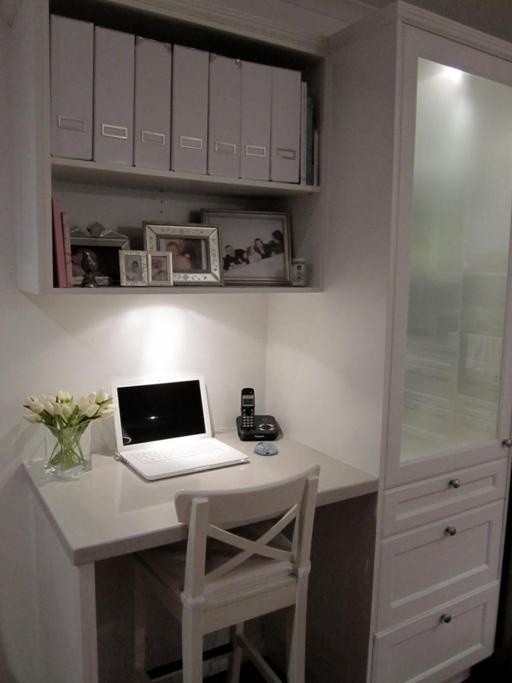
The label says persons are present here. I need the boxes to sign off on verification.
[223,230,284,270]
[168,242,191,269]
[127,260,140,279]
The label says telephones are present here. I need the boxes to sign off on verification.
[236,388,279,441]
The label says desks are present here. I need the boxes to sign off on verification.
[21,430,379,683]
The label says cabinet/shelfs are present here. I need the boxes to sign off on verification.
[17,1,331,295]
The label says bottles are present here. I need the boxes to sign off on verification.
[292,258,306,287]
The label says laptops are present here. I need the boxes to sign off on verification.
[111,375,249,482]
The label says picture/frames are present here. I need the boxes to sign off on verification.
[118,249,148,287]
[142,222,224,288]
[69,221,131,287]
[201,208,293,285]
[148,250,173,287]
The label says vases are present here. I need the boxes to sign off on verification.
[43,423,94,475]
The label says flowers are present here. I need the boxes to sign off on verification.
[25,390,116,469]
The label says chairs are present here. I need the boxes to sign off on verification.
[129,464,323,683]
